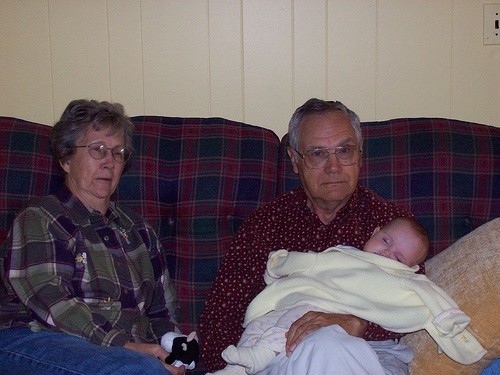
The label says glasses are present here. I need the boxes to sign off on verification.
[73,142,131,163]
[288,144,361,169]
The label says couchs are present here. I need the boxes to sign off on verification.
[0,116,500,375]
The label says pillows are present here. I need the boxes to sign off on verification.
[368,217,500,375]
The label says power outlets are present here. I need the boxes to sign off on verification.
[483,3,500,44]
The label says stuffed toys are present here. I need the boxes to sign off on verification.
[161,331,199,370]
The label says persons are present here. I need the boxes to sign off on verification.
[197,98,425,375]
[0,99,186,375]
[205,217,430,375]
